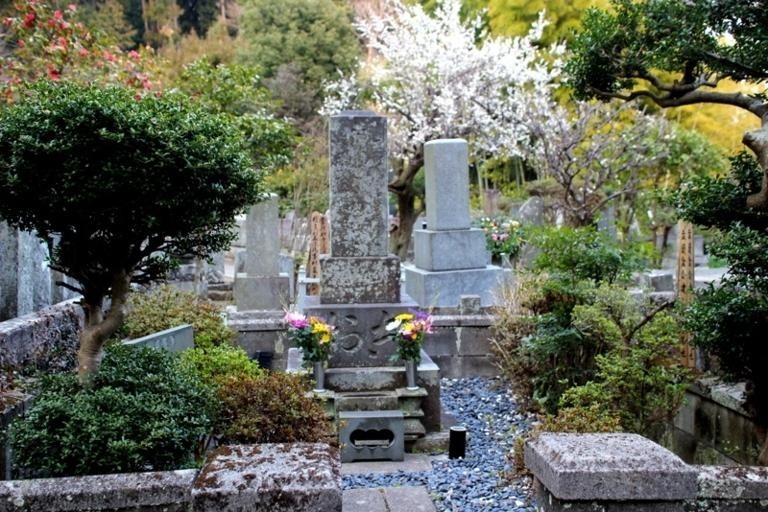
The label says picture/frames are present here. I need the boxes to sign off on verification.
[404,360,420,391]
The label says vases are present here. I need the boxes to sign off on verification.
[312,361,328,394]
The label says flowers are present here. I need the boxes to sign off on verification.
[279,306,337,370]
[382,313,434,366]
